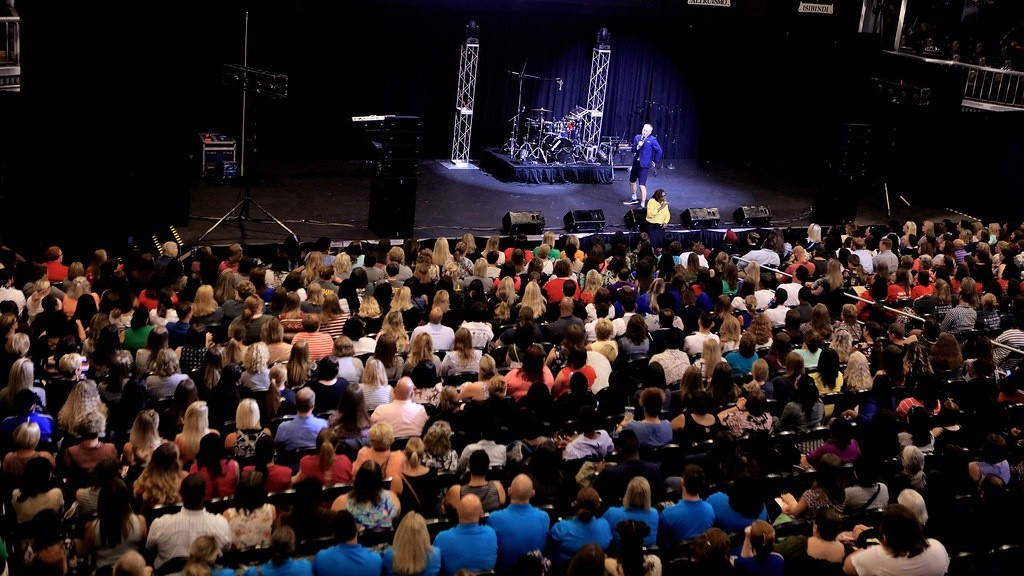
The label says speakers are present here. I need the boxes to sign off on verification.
[624,209,646,229]
[732,204,773,224]
[680,208,720,229]
[502,210,545,235]
[563,208,606,233]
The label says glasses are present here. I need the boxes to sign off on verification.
[662,193,666,198]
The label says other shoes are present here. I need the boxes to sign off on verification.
[623,197,639,205]
[637,204,645,209]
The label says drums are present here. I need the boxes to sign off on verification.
[536,121,553,133]
[540,134,574,164]
[554,121,571,133]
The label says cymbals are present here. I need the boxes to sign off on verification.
[531,108,551,112]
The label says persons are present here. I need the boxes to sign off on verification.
[623,124,662,209]
[0,218,1024,575]
[902,18,1024,105]
[645,189,670,251]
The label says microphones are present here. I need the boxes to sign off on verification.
[559,80,562,90]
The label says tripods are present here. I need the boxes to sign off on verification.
[515,106,549,166]
[196,12,301,242]
